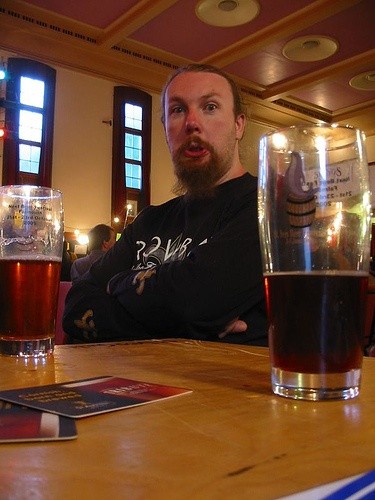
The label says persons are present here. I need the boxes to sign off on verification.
[60,64,337,347]
[71,224,115,280]
[43,238,77,282]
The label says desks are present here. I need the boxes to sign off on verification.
[0,338,375,500]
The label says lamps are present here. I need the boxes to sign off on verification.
[349,71,375,91]
[282,35,340,63]
[195,0,261,28]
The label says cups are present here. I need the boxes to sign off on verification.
[0,185,64,357]
[256,124,370,402]
